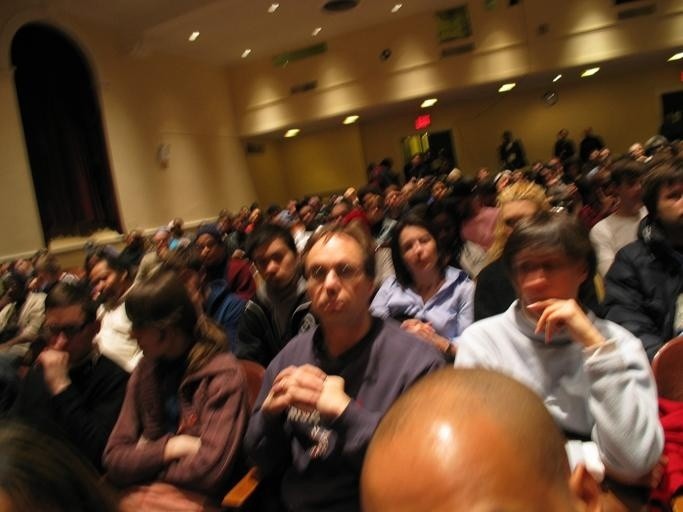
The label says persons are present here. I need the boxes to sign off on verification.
[1,124,681,511]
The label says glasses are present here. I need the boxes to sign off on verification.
[44,319,87,336]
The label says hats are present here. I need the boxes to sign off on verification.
[194,224,221,244]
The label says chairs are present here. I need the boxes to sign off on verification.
[222,361,267,507]
[649,337,683,398]
